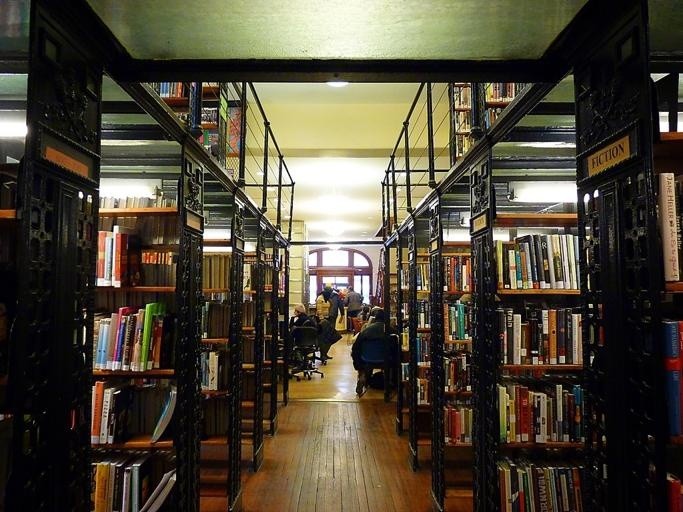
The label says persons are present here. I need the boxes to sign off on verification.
[288,280,398,375]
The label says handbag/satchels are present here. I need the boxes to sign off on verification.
[337,316,346,330]
[369,369,384,390]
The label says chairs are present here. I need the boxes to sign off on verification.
[355,329,400,401]
[290,326,325,381]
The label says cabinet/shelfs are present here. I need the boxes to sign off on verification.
[203,180,243,512]
[152,81,201,140]
[92,140,202,511]
[572,0,682,510]
[407,222,428,476]
[393,236,407,437]
[201,81,227,180]
[428,191,469,512]
[469,152,582,512]
[264,234,278,434]
[472,82,529,135]
[278,243,290,406]
[244,217,264,473]
[446,83,471,165]
[0,0,104,512]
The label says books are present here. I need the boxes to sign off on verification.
[388,79,683,512]
[0,80,286,512]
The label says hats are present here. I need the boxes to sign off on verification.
[324,287,333,293]
[295,303,306,312]
